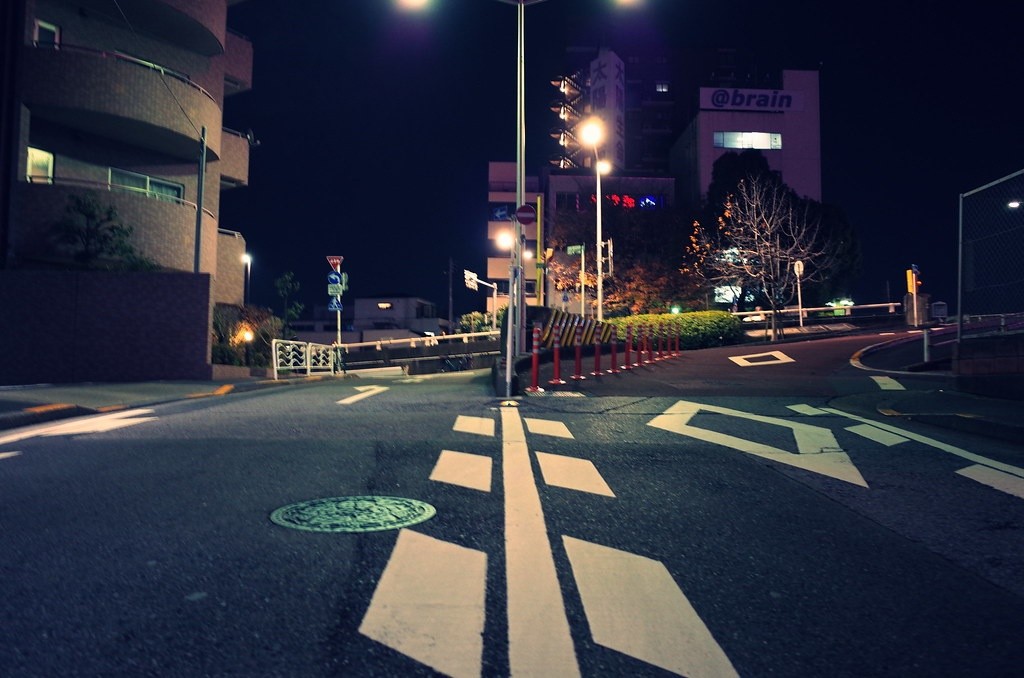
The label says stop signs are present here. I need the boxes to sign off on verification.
[515,204,536,230]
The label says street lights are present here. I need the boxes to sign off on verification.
[582,121,605,323]
[241,253,251,307]
[400,0,642,352]
